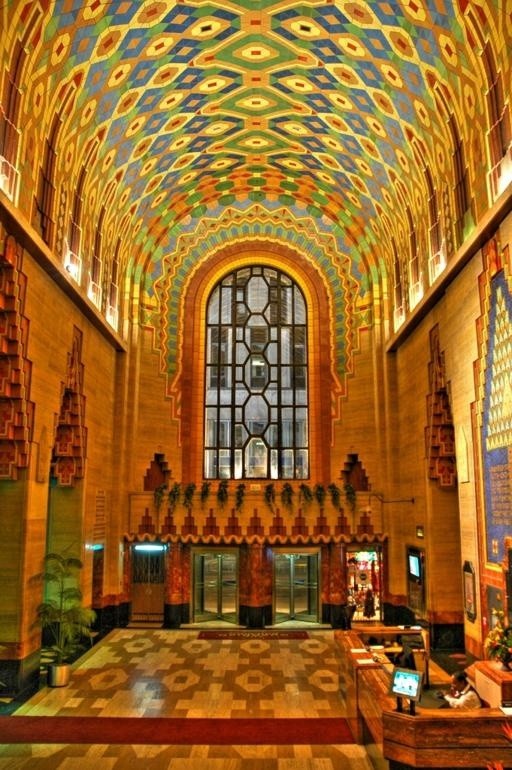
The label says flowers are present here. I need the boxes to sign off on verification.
[483,607,512,663]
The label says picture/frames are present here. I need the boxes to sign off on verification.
[463,560,477,623]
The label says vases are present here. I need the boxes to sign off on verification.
[496,653,512,671]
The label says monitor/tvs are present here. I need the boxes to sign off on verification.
[408,548,423,584]
[387,668,424,701]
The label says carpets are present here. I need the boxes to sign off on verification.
[197,630,310,640]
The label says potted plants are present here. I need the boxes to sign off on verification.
[26,539,98,688]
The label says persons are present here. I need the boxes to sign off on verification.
[442,672,483,709]
